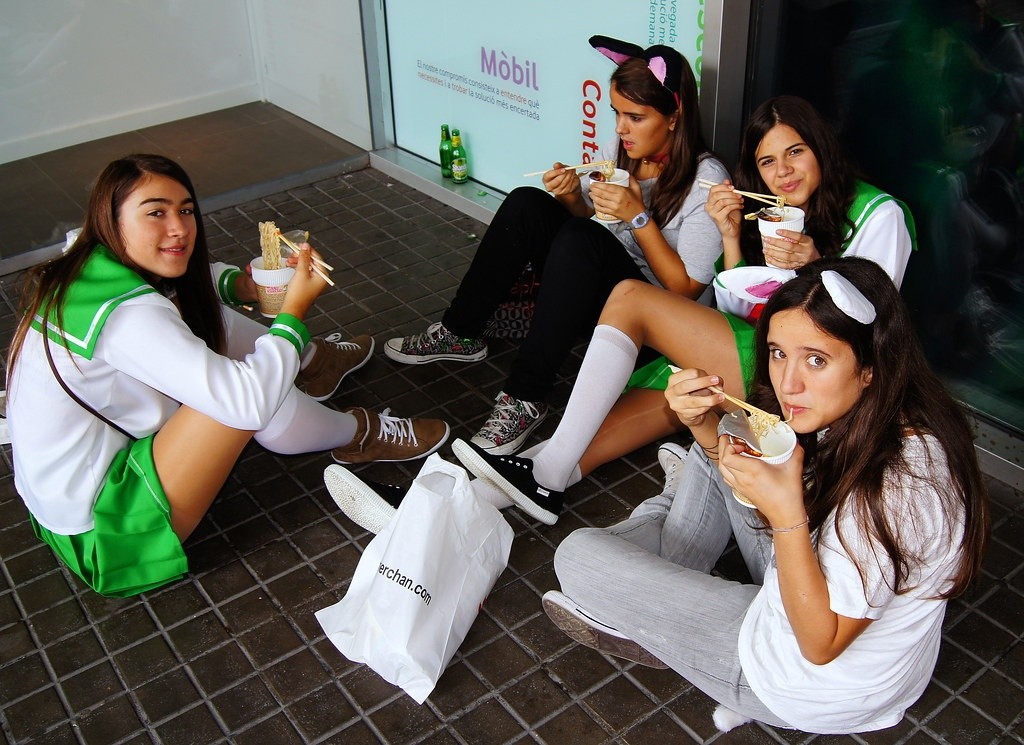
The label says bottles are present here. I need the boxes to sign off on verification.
[439,124,453,178]
[449,129,468,184]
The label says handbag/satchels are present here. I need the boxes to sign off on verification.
[482,263,540,339]
[316,453,516,705]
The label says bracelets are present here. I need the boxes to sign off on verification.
[773,516,809,533]
[697,442,719,460]
[629,210,650,230]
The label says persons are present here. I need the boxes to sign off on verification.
[4,153,450,599]
[875,0,1024,346]
[541,257,985,735]
[384,35,730,454]
[325,97,917,536]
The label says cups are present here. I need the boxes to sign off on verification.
[588,169,631,224]
[759,206,805,266]
[731,416,797,508]
[250,257,295,315]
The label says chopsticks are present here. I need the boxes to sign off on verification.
[668,365,780,426]
[698,178,782,207]
[277,234,336,288]
[524,160,610,178]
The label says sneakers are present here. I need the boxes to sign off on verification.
[470,391,549,455]
[657,443,689,502]
[383,322,488,364]
[324,464,408,535]
[541,590,670,669]
[451,437,566,525]
[331,407,450,464]
[300,333,375,400]
